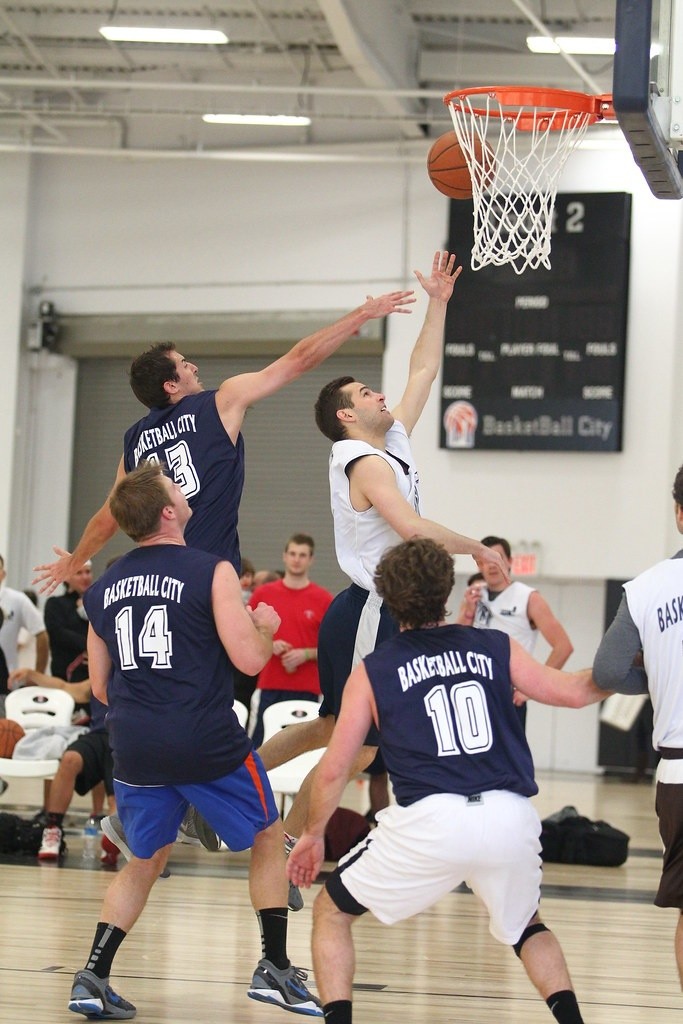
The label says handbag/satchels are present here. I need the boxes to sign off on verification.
[540,803,631,867]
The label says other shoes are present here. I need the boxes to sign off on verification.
[82,822,98,837]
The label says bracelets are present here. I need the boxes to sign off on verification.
[465,610,474,619]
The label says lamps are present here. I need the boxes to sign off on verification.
[93,12,228,46]
[201,99,315,127]
[523,15,663,59]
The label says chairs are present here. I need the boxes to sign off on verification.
[260,698,332,821]
[229,698,250,733]
[0,687,76,780]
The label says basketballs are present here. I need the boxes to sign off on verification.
[427,129,499,201]
[0,718,25,759]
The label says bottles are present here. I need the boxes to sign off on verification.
[82,813,99,864]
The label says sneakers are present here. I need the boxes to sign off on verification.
[99,834,120,867]
[285,840,304,911]
[174,817,198,842]
[39,826,61,859]
[66,968,137,1019]
[247,959,322,1015]
[99,813,170,879]
[193,805,222,851]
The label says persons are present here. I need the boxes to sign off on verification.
[592,465,683,1000]
[68,467,325,1021]
[457,536,574,738]
[0,556,126,865]
[192,250,512,911]
[285,538,644,1024]
[467,572,487,587]
[239,534,389,822]
[32,291,416,879]
